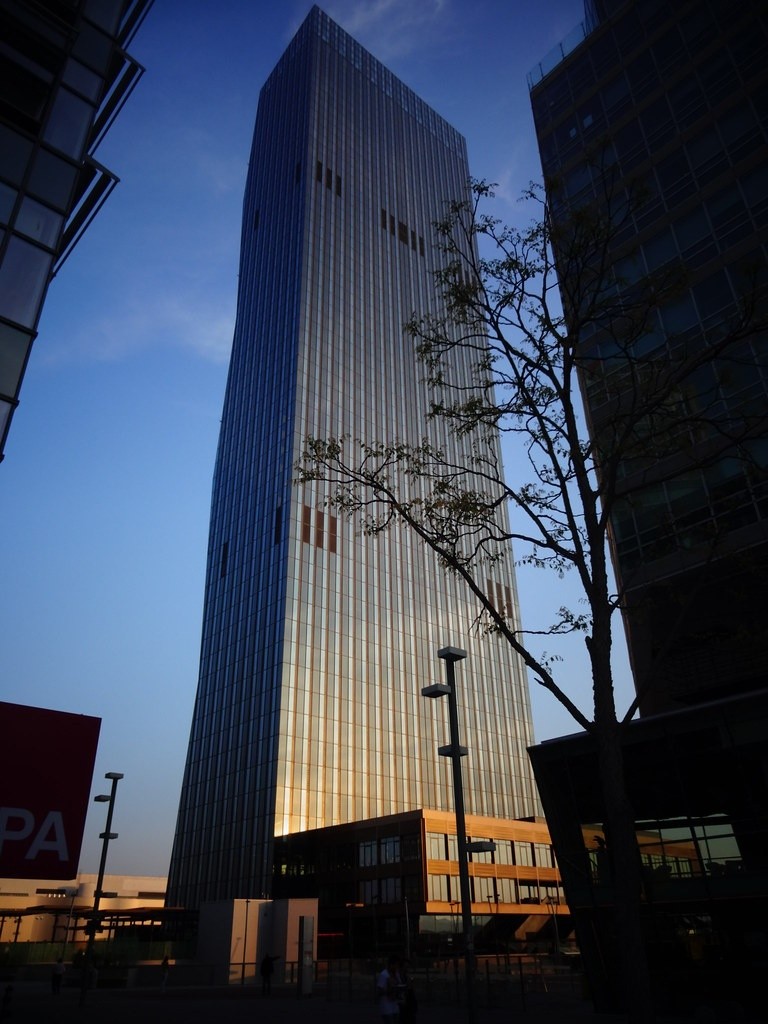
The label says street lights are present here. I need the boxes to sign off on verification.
[59,888,80,968]
[371,894,383,973]
[240,898,250,984]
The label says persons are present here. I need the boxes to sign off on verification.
[51,958,66,995]
[260,953,281,997]
[159,955,169,990]
[377,955,418,1024]
[302,951,313,1000]
